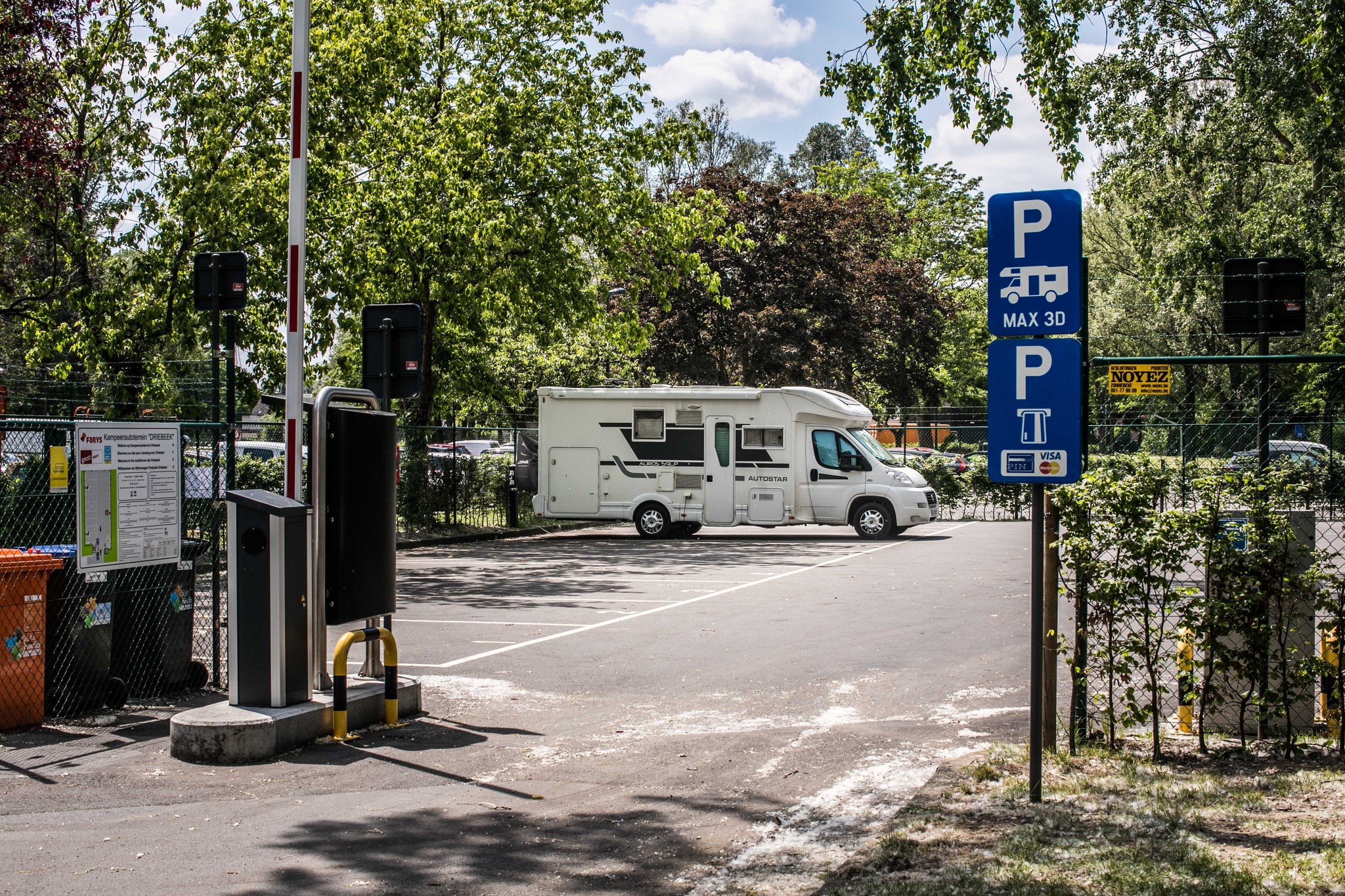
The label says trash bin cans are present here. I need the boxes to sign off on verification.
[1203,510,1315,735]
[111,537,209,696]
[225,489,312,707]
[15,545,128,718]
[0,547,64,733]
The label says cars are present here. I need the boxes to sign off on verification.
[1215,438,1345,505]
[884,446,988,483]
[399,439,515,489]
[184,439,309,469]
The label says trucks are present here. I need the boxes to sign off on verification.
[511,383,939,541]
